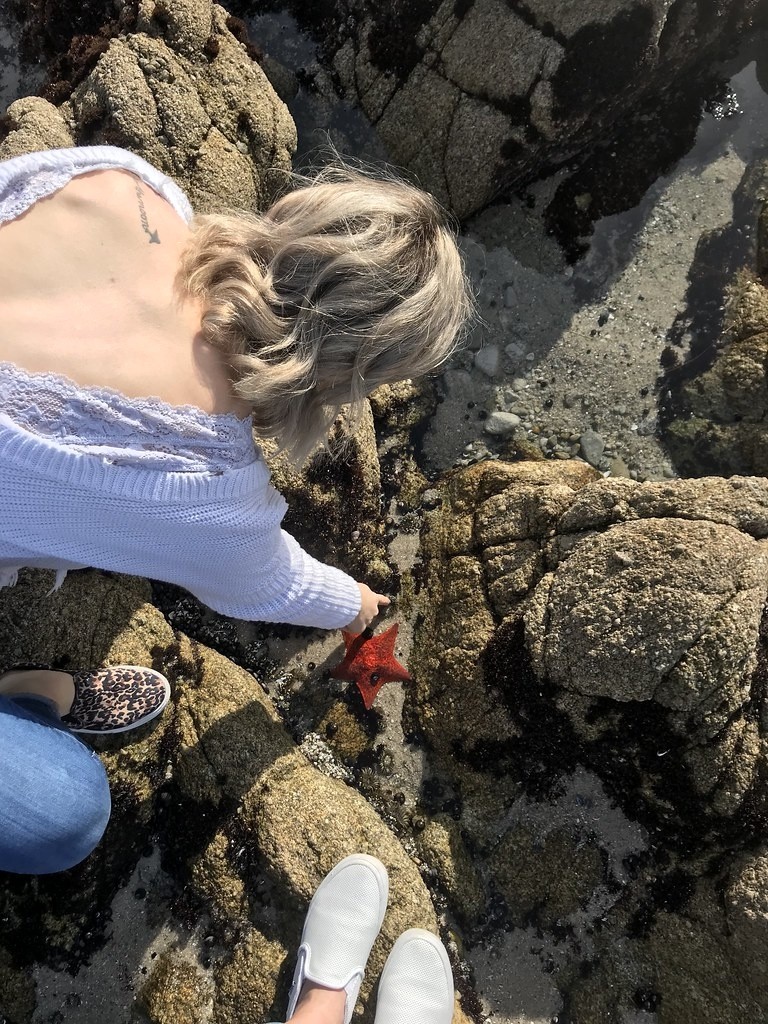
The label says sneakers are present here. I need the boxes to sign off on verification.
[286,853,389,1024]
[0,665,171,733]
[373,928,455,1024]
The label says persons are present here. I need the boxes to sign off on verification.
[284,852,453,1024]
[0,146,489,872]
